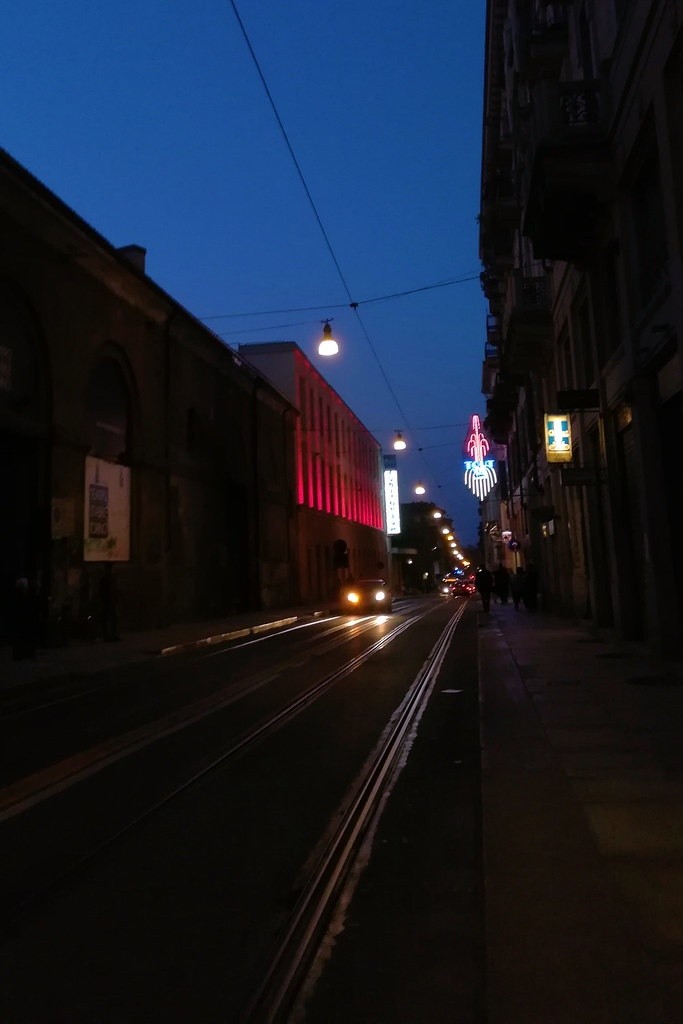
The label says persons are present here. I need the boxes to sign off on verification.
[13,577,38,660]
[475,563,493,611]
[512,564,540,613]
[494,561,510,604]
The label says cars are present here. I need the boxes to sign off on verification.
[347,579,392,615]
[441,577,477,598]
